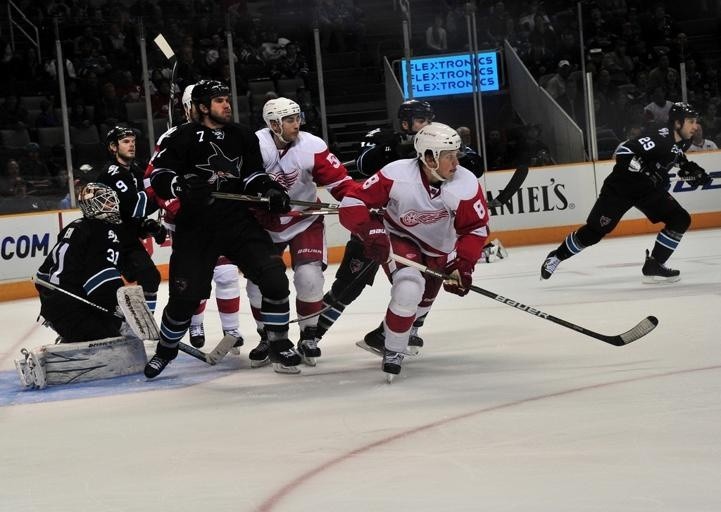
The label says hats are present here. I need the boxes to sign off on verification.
[558,60,570,68]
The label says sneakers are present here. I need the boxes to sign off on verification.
[223,329,243,348]
[480,248,489,262]
[382,348,405,374]
[20,348,47,389]
[409,325,423,346]
[483,242,502,258]
[188,323,204,348]
[13,358,34,390]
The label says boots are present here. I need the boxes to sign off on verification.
[142,355,168,379]
[541,250,563,280]
[642,249,680,276]
[364,321,385,353]
[247,324,322,375]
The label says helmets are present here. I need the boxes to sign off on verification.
[262,97,305,126]
[413,121,462,154]
[397,100,436,120]
[78,183,122,224]
[181,85,195,111]
[181,85,194,106]
[668,103,702,122]
[191,79,231,107]
[105,126,136,147]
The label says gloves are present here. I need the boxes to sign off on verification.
[359,221,391,264]
[173,173,211,204]
[443,256,476,297]
[139,217,166,245]
[644,167,670,189]
[264,188,290,215]
[678,162,712,189]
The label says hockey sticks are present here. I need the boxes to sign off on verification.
[213,165,529,216]
[391,254,659,345]
[31,275,236,366]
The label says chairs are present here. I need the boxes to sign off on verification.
[1,0,721,159]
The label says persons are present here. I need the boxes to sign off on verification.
[541,103,712,285]
[316,100,490,380]
[93,126,166,313]
[1,0,720,216]
[142,80,243,355]
[249,96,353,369]
[144,81,302,379]
[34,183,161,344]
